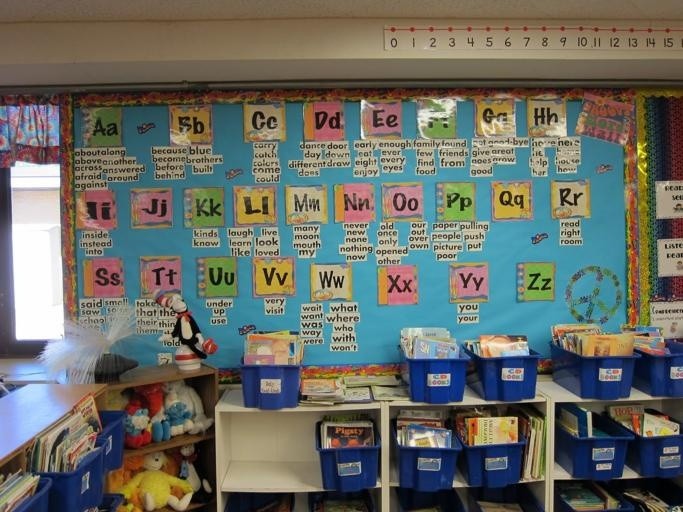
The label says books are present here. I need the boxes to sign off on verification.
[299,374,412,407]
[244,330,304,365]
[0,394,103,512]
[397,403,547,481]
[465,335,530,358]
[320,413,375,449]
[551,324,672,358]
[400,328,460,359]
[479,501,523,512]
[559,403,683,512]
[313,491,368,512]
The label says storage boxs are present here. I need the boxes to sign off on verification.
[4,407,128,512]
[212,335,682,511]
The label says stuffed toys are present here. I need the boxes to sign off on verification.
[154,288,217,371]
[108,379,213,512]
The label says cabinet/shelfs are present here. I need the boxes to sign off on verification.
[0,381,114,511]
[107,357,220,511]
